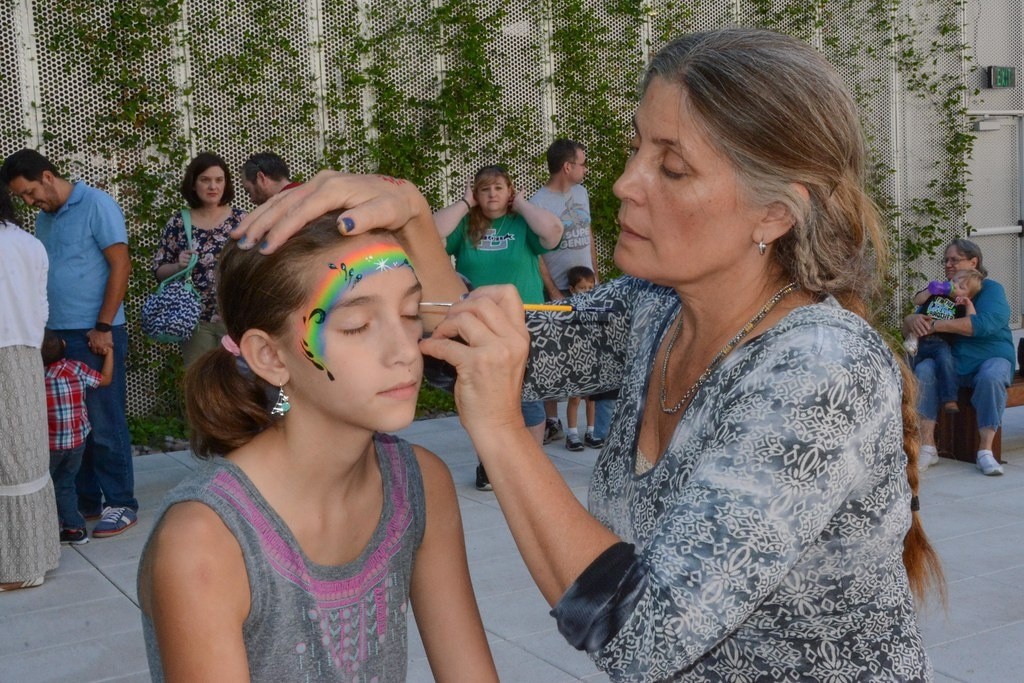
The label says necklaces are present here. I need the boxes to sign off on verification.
[489,216,507,241]
[659,283,802,414]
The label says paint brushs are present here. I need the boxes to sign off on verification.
[419,302,572,311]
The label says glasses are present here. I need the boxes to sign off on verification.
[566,159,587,169]
[941,255,972,267]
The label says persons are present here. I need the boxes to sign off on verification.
[240,151,305,206]
[0,178,61,591]
[152,151,248,368]
[230,28,935,683]
[433,140,617,490]
[3,149,139,544]
[136,208,501,683]
[901,239,1024,475]
[41,336,113,543]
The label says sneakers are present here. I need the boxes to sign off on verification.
[917,444,939,472]
[566,432,584,451]
[543,417,564,444]
[60,526,89,545]
[944,400,959,414]
[81,504,103,521]
[93,505,138,537]
[476,461,494,491]
[584,434,604,449]
[977,454,1004,476]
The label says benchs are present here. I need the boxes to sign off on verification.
[934,374,1024,465]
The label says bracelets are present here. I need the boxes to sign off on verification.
[462,197,471,212]
[95,321,113,332]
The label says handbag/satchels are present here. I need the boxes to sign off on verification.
[139,208,203,343]
